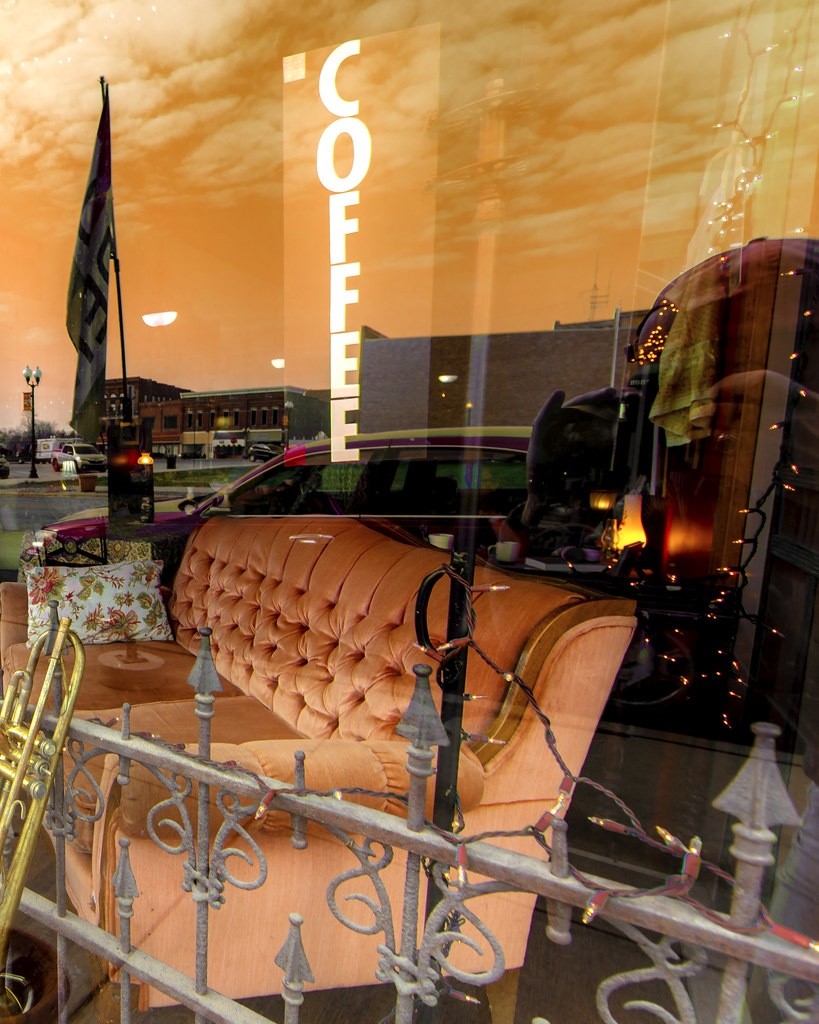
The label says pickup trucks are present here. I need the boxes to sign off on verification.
[51,444,107,473]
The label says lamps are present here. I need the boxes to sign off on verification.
[138,453,155,465]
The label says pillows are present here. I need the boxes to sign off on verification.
[23,559,174,650]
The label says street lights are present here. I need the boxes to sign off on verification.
[23,365,42,478]
[467,400,472,431]
[285,401,293,451]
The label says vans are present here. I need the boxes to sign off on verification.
[36,438,85,464]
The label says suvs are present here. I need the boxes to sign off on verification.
[248,444,284,463]
[20,427,535,565]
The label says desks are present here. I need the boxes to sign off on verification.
[17,516,205,582]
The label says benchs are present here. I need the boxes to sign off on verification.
[0,515,639,1024]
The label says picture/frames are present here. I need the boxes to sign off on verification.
[119,415,141,448]
